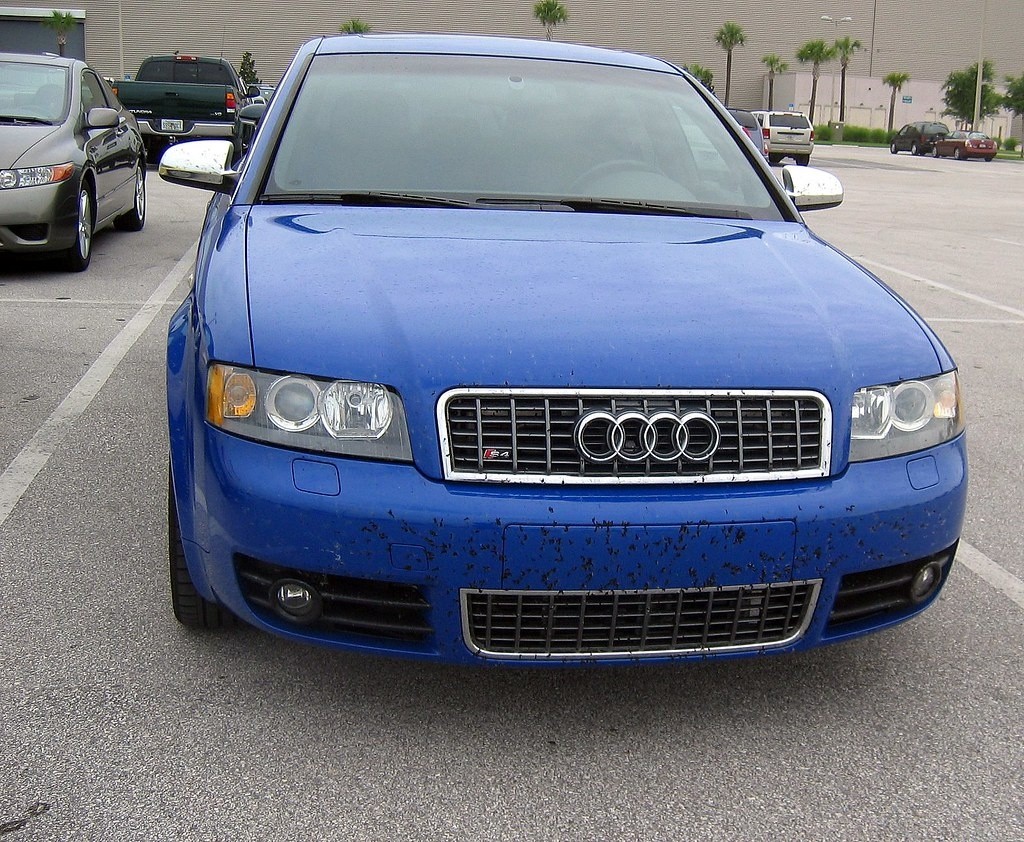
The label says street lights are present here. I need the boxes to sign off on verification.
[820,16,852,121]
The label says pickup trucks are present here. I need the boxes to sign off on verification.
[103,55,261,165]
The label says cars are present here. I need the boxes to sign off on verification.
[930,130,997,162]
[247,84,276,105]
[890,121,949,156]
[726,108,769,164]
[0,52,148,273]
[751,110,814,167]
[158,32,969,667]
